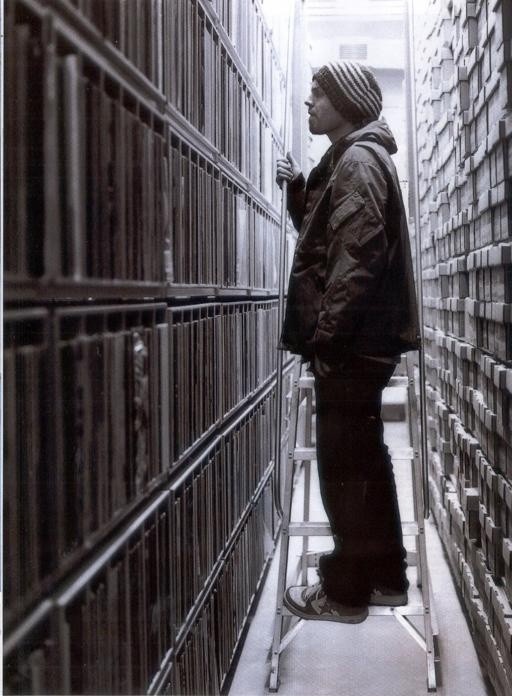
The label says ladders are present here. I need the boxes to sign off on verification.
[268,352,438,694]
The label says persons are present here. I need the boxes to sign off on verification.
[276,58,423,625]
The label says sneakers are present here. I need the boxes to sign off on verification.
[282,583,368,624]
[371,585,410,608]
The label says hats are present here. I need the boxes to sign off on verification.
[312,61,383,122]
